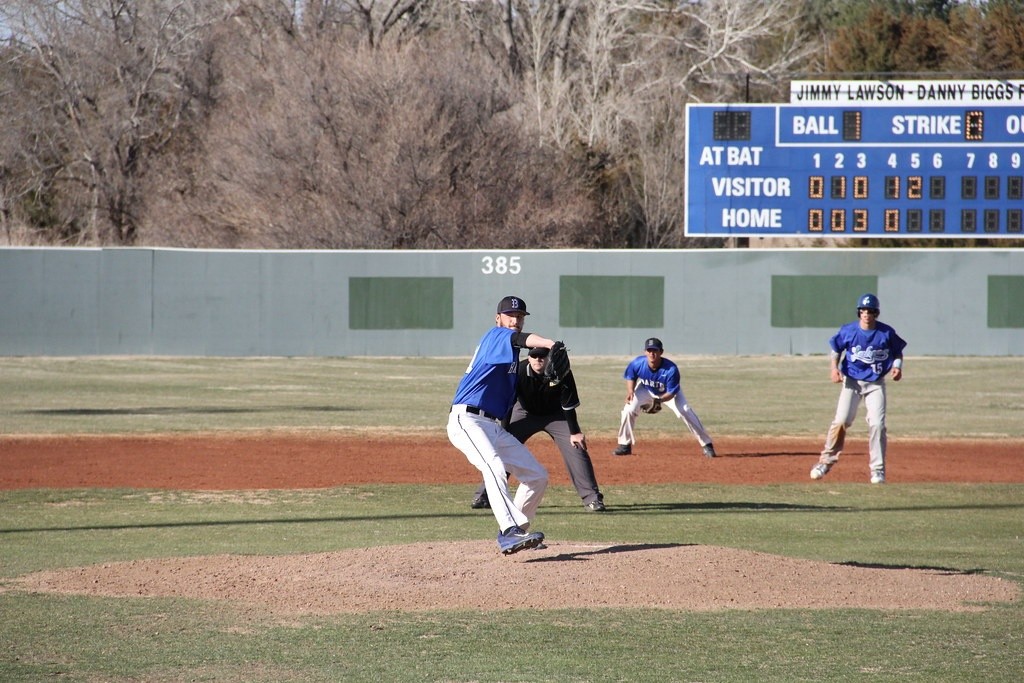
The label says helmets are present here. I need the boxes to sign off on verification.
[857,293,879,310]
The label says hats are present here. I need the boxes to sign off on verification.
[528,348,549,357]
[644,338,662,351]
[497,296,531,316]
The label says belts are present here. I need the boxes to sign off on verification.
[449,404,505,428]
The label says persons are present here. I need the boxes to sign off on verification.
[612,338,717,457]
[446,294,570,554]
[809,294,908,483]
[470,347,605,511]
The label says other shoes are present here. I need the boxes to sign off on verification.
[703,447,715,457]
[613,446,631,455]
[809,461,833,480]
[870,472,885,484]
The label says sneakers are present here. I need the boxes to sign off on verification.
[497,530,546,549]
[497,527,544,555]
[588,501,605,511]
[470,499,490,509]
